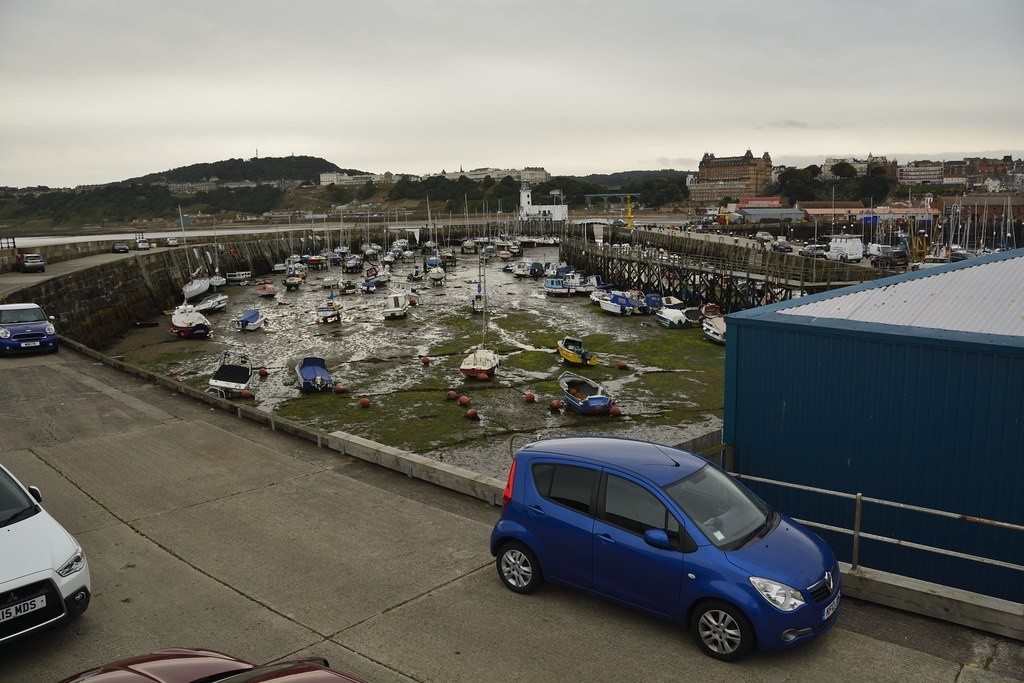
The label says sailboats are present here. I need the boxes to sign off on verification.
[317,237,344,317]
[460,255,500,377]
[178,194,559,315]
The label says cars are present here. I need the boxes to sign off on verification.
[134,239,149,250]
[59,648,367,682]
[756,231,976,271]
[613,218,641,227]
[0,303,58,356]
[15,254,45,273]
[0,463,90,646]
[489,436,841,662]
[163,238,179,247]
[111,242,129,253]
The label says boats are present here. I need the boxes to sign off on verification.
[557,336,597,365]
[171,299,212,339]
[209,350,253,396]
[501,260,727,344]
[231,308,267,331]
[558,370,614,415]
[295,357,334,393]
